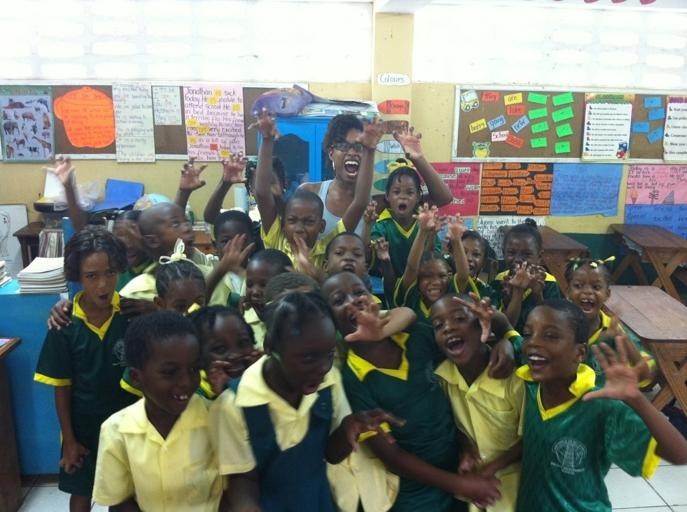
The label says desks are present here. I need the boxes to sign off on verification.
[602,285,686,423]
[182,221,211,254]
[494,224,589,300]
[2,264,106,479]
[14,222,88,267]
[0,334,22,506]
[609,221,687,304]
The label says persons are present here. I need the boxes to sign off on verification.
[30,107,685,511]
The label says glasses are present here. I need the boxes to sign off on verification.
[330,142,363,152]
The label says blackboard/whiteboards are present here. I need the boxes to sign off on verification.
[1,205,31,277]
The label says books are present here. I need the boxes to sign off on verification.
[0,258,13,290]
[17,257,67,294]
